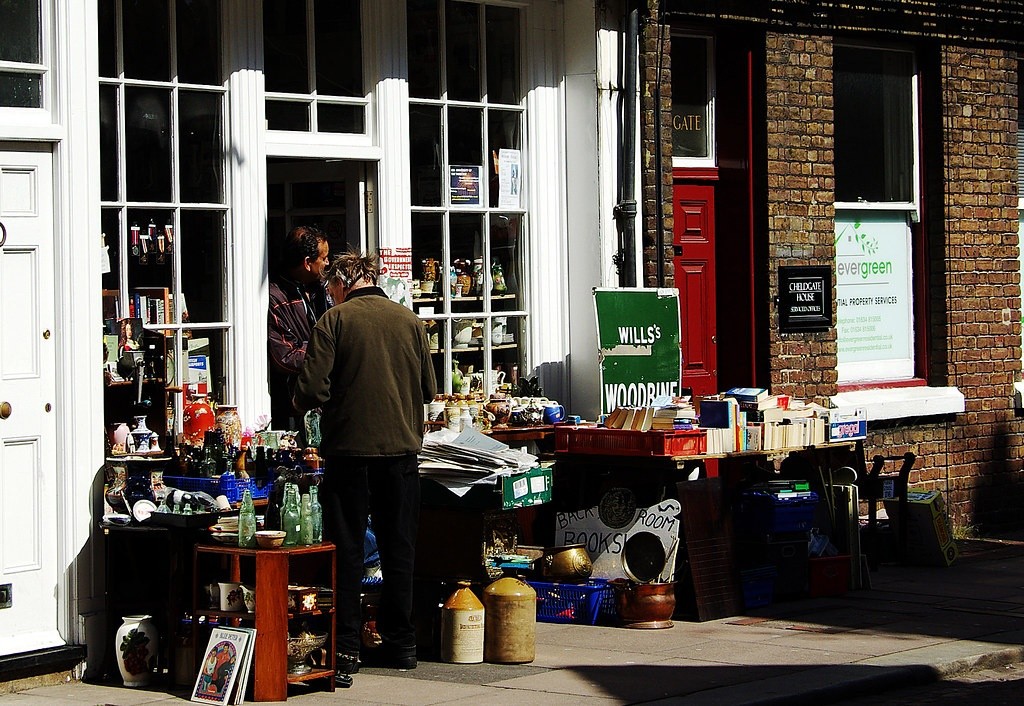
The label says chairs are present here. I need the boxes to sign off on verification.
[854,452,917,572]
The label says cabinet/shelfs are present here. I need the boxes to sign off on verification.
[195,541,337,702]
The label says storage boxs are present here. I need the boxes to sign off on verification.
[883,488,959,568]
[556,426,708,455]
[819,407,867,443]
[528,580,621,626]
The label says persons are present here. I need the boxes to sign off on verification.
[119,319,140,351]
[268,223,437,669]
[118,318,145,352]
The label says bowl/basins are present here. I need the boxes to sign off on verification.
[239,585,256,613]
[608,578,676,629]
[548,542,593,582]
[254,531,286,548]
[621,530,666,582]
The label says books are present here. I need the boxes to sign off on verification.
[604,388,826,456]
[129,286,174,325]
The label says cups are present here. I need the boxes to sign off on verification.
[510,397,565,428]
[218,582,243,612]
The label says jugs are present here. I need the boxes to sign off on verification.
[479,370,505,394]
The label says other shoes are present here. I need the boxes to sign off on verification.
[308,638,362,673]
[364,645,418,668]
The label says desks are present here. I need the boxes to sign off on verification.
[99,475,521,685]
[538,440,860,624]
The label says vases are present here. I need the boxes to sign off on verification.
[548,543,593,585]
[606,580,678,629]
[116,615,159,687]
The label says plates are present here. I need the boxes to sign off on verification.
[211,533,239,545]
[133,500,157,521]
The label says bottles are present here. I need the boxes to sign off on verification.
[165,432,318,486]
[441,580,484,663]
[183,504,192,515]
[281,483,322,546]
[158,500,167,513]
[484,567,536,664]
[173,505,180,514]
[433,393,484,433]
[238,488,257,547]
